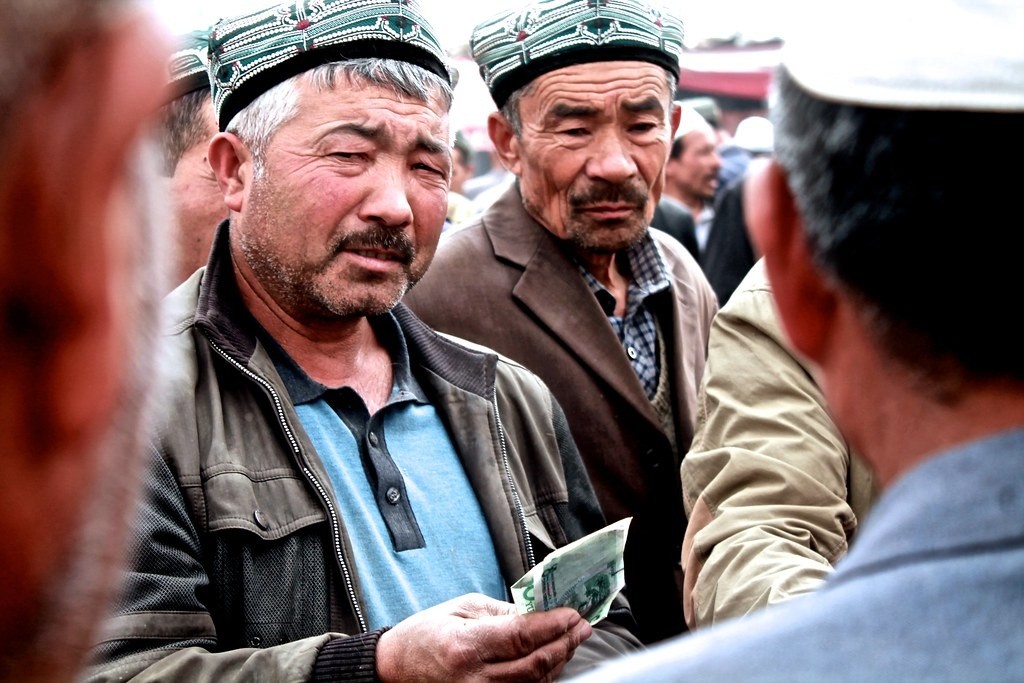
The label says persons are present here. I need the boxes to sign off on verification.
[651,97,772,308]
[398,0,722,649]
[566,15,1024,683]
[679,252,881,634]
[119,18,229,304]
[440,127,516,233]
[63,0,644,683]
[0,1,178,682]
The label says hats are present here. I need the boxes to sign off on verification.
[733,116,776,151]
[469,0,684,108]
[667,99,712,141]
[768,0,1024,117]
[150,28,213,112]
[207,0,450,131]
